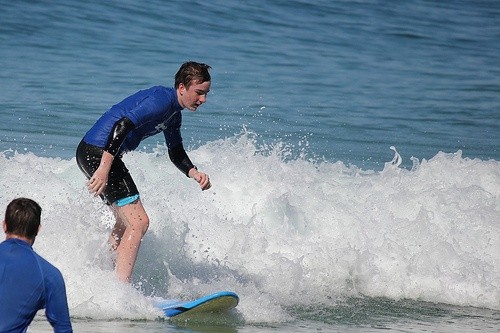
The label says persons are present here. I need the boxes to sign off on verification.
[76,61,213,284]
[0,197,73,333]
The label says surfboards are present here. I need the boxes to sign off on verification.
[149,292,240,319]
